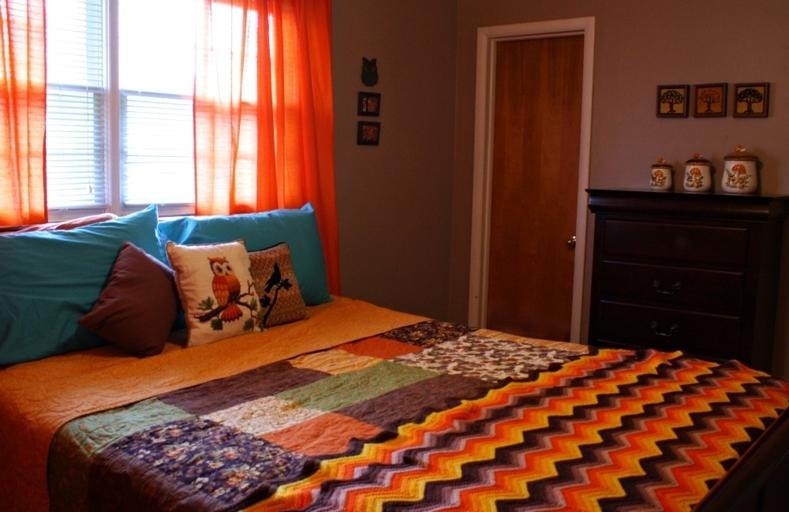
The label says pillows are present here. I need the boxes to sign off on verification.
[165,239,268,348]
[0,202,169,365]
[74,240,183,358]
[15,212,119,233]
[158,201,335,305]
[247,241,311,328]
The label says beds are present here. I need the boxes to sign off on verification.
[0,294,789,512]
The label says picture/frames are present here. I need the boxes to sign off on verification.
[357,91,381,116]
[656,84,690,119]
[733,82,770,118]
[357,120,381,145]
[692,82,728,118]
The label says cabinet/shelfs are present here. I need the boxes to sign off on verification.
[584,187,789,373]
